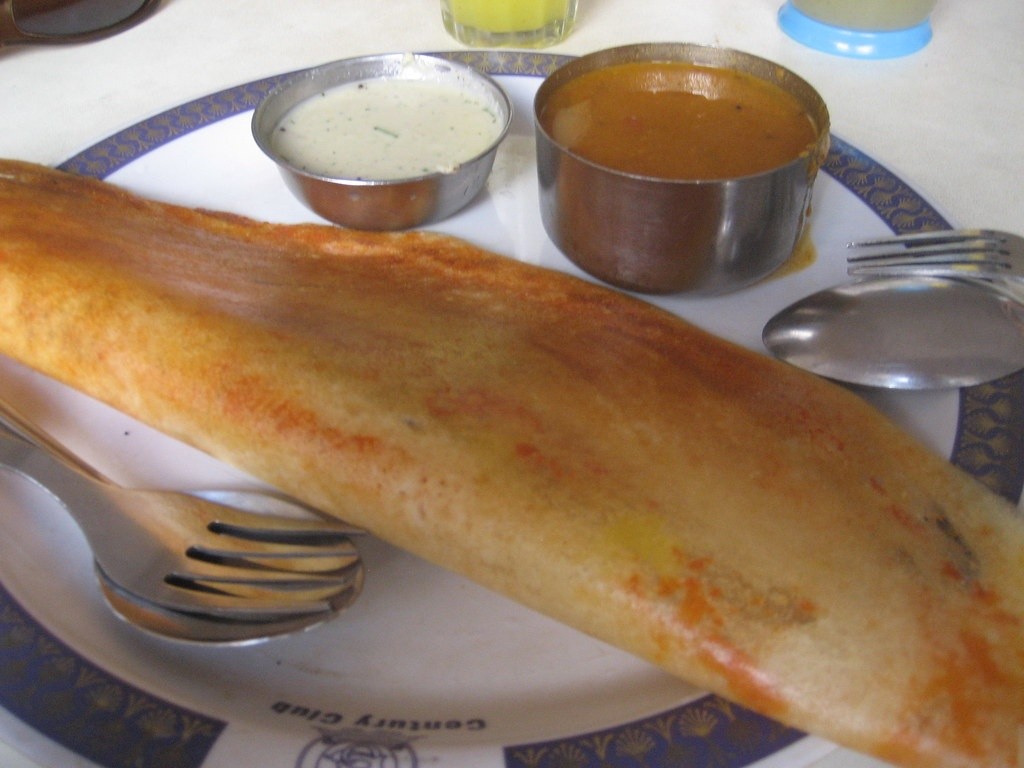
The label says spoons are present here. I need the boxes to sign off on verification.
[762,275,1024,390]
[0,400,366,646]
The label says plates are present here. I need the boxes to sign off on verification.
[0,52,1024,768]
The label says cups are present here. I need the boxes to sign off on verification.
[777,0,939,58]
[440,0,577,50]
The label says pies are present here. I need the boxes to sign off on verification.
[1,156,1024,768]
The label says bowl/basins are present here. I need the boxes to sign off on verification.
[532,43,830,297]
[252,53,513,231]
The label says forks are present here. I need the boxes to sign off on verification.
[843,225,1024,280]
[0,427,366,617]
